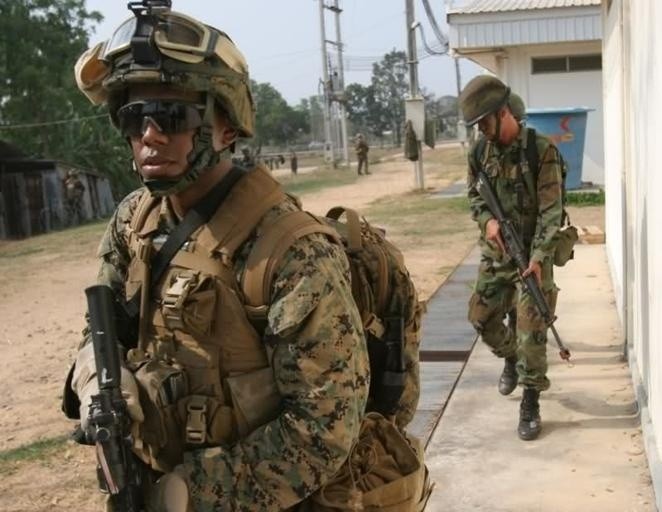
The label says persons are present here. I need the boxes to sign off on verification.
[64,168,89,225]
[355,132,372,176]
[461,72,564,442]
[240,145,255,166]
[289,147,298,175]
[61,0,436,511]
[505,91,569,334]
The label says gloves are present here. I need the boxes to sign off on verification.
[149,473,192,511]
[79,366,145,440]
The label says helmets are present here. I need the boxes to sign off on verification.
[101,14,253,139]
[457,76,511,127]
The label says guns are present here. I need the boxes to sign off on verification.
[85,284,142,512]
[472,171,571,364]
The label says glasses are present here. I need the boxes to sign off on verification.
[102,12,210,65]
[115,98,207,139]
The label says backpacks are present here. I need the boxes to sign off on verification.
[239,206,421,429]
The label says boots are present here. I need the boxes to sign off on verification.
[498,355,518,395]
[519,390,540,440]
